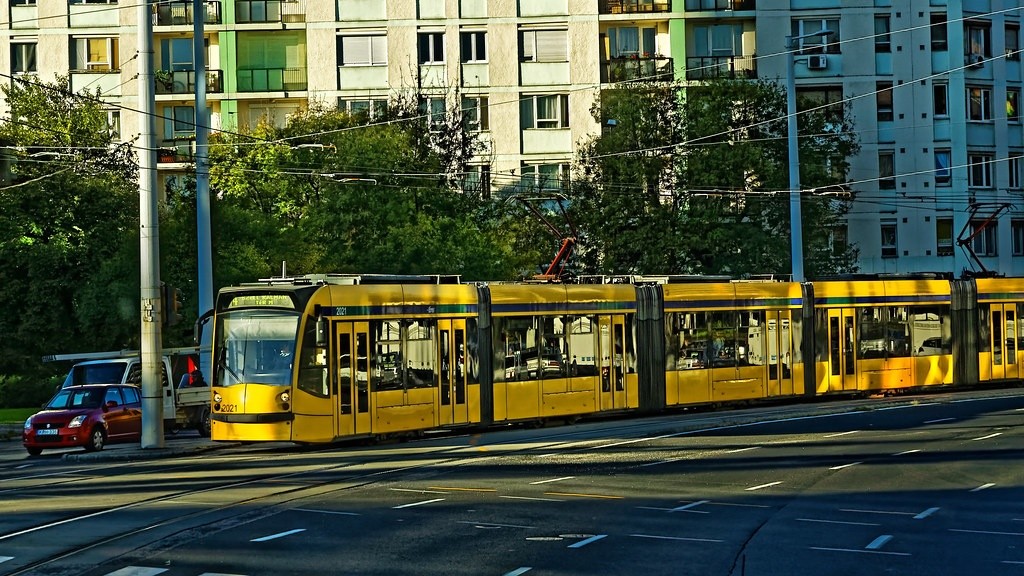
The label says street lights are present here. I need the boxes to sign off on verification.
[785,30,837,284]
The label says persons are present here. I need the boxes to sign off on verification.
[190,368,208,388]
[272,341,295,371]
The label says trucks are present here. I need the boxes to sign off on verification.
[58,354,212,439]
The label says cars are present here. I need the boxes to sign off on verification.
[21,383,142,456]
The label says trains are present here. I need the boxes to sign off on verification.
[191,255,1024,443]
